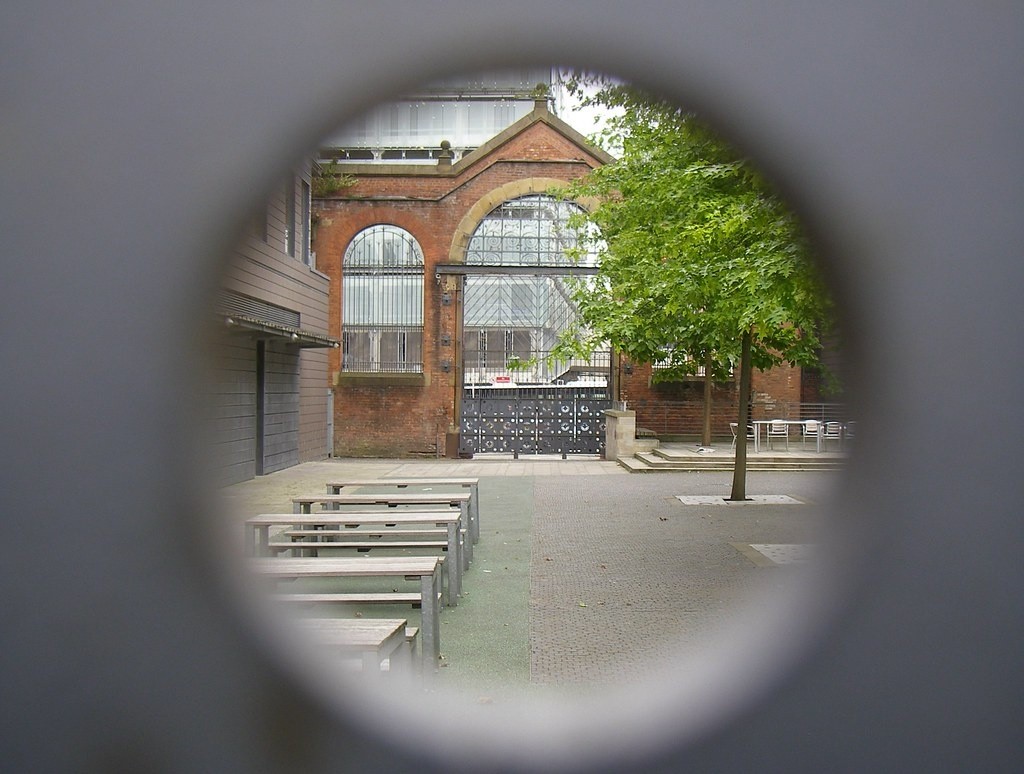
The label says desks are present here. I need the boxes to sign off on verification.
[282,618,409,702]
[752,421,822,453]
[325,478,480,546]
[292,493,474,569]
[240,554,441,680]
[246,511,462,606]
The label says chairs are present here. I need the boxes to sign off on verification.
[767,420,789,451]
[824,422,843,452]
[844,421,856,452]
[730,423,754,453]
[803,420,824,451]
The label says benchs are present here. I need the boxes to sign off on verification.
[257,502,479,670]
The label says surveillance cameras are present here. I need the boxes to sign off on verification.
[435,274,442,279]
